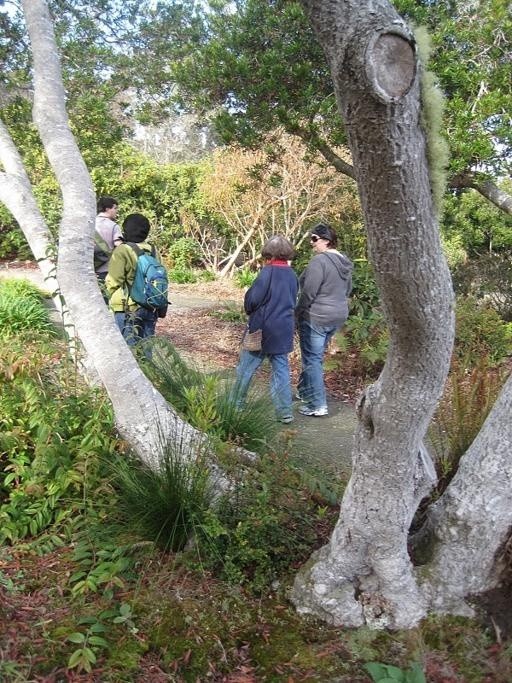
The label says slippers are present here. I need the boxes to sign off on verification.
[119,242,168,311]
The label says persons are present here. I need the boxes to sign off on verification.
[104,214,165,368]
[94,198,125,284]
[295,221,358,419]
[228,230,299,423]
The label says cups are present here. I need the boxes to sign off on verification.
[310,235,323,242]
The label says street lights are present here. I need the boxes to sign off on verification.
[242,328,262,351]
[94,230,112,268]
[158,305,167,318]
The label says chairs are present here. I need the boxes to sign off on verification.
[295,391,328,416]
[273,414,294,423]
